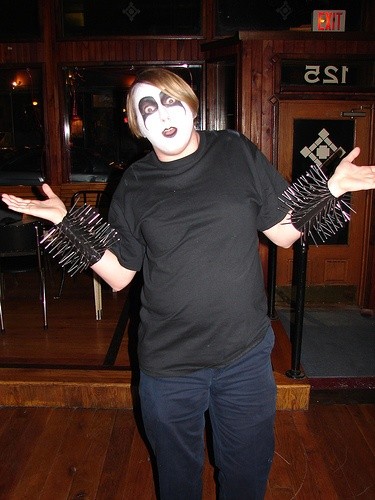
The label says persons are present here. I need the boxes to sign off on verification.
[1,68,375,500]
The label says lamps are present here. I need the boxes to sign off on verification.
[71,114,82,137]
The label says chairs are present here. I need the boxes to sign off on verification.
[0,190,108,335]
